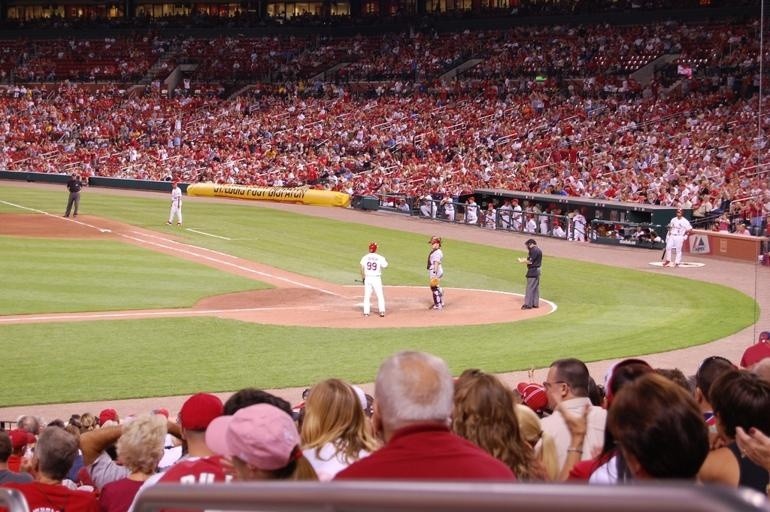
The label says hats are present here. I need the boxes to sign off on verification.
[526,239,535,244]
[100,409,116,426]
[7,430,27,448]
[205,403,301,471]
[429,236,440,244]
[181,394,224,429]
[517,383,548,410]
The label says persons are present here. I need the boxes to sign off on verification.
[517,239,542,309]
[1,331,770,511]
[1,0,769,265]
[425,235,445,309]
[360,243,388,318]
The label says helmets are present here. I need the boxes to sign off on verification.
[369,244,376,253]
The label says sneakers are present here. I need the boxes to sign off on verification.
[433,304,441,309]
[521,305,530,309]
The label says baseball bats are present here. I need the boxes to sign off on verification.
[661,249,665,260]
[354,279,363,283]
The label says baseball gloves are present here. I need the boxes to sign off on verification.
[430,277,440,288]
